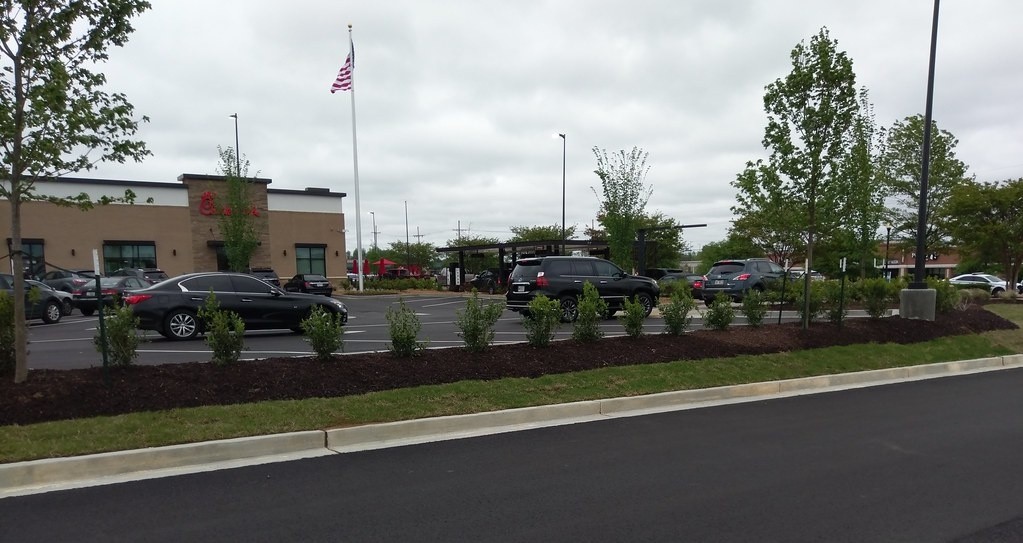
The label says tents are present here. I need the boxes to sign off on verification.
[373,258,396,272]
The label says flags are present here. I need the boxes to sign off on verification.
[330,40,355,93]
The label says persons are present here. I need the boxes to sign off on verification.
[399,265,421,275]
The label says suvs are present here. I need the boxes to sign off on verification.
[41,270,104,317]
[244,267,281,290]
[114,267,170,286]
[704,258,798,309]
[506,256,661,323]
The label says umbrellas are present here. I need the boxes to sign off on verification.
[353,258,357,274]
[363,259,369,274]
[379,257,386,278]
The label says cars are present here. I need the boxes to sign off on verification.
[121,271,347,340]
[938,273,1023,298]
[283,274,332,296]
[0,273,73,323]
[470,268,510,295]
[74,274,154,316]
[655,273,703,300]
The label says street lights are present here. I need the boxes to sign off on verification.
[230,112,240,176]
[884,222,892,281]
[559,133,566,255]
